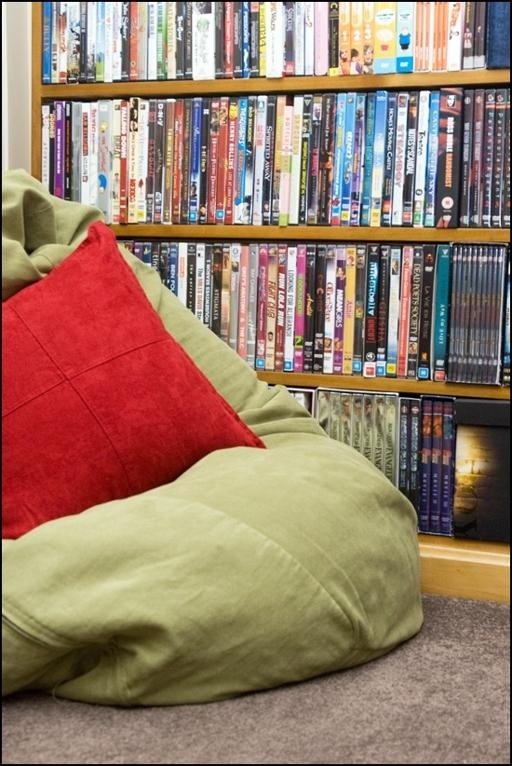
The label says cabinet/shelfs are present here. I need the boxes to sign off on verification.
[30,0,509,603]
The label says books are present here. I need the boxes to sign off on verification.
[122,242,512,377]
[287,390,512,544]
[38,87,509,229]
[43,1,511,83]
[113,238,511,389]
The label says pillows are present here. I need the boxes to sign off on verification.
[0,216,266,543]
